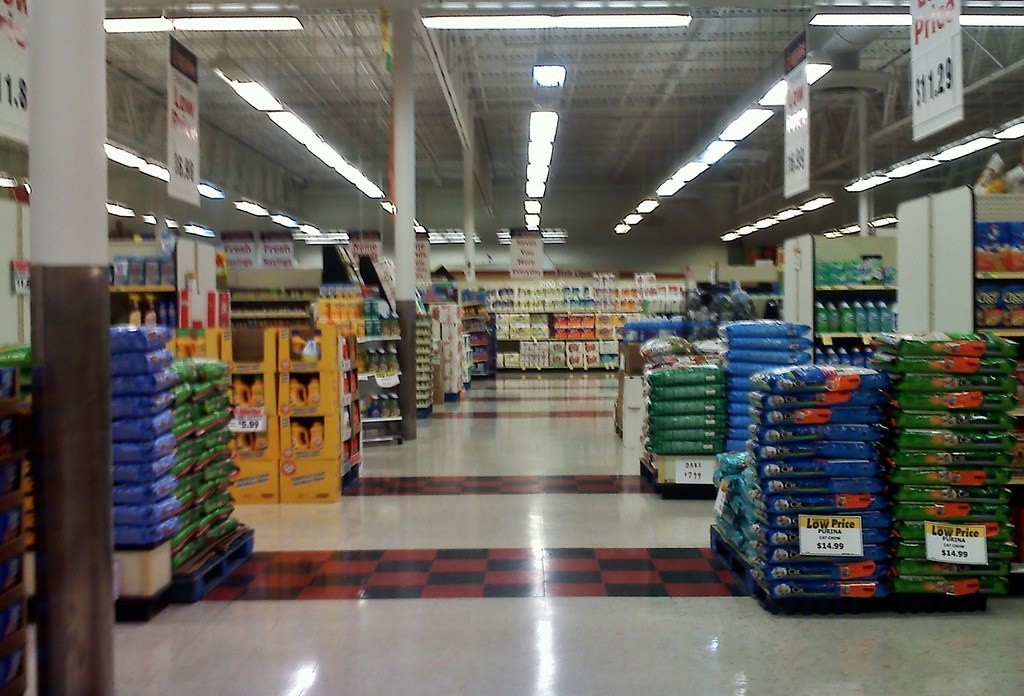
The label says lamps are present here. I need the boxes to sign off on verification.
[104,15,304,32]
[524,112,559,231]
[421,15,692,28]
[808,12,1024,28]
[614,0,832,235]
[532,51,566,90]
[844,91,1024,191]
[211,54,385,199]
[720,194,835,241]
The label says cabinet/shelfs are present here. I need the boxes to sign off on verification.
[974,270,1024,576]
[416,313,434,420]
[228,286,403,443]
[465,315,498,381]
[814,284,898,338]
[488,312,683,370]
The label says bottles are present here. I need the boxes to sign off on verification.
[307,378,321,408]
[313,330,321,348]
[287,374,307,408]
[317,282,401,418]
[126,292,176,326]
[251,376,264,407]
[302,340,319,362]
[816,347,876,367]
[815,300,896,333]
[290,329,306,363]
[290,421,309,452]
[309,421,325,453]
[231,375,251,408]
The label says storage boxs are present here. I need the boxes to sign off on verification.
[167,324,364,503]
[428,305,466,393]
[611,340,642,433]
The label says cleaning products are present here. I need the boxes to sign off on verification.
[290,421,325,450]
[815,346,875,369]
[289,376,320,407]
[234,429,269,452]
[232,374,265,408]
[814,299,898,334]
